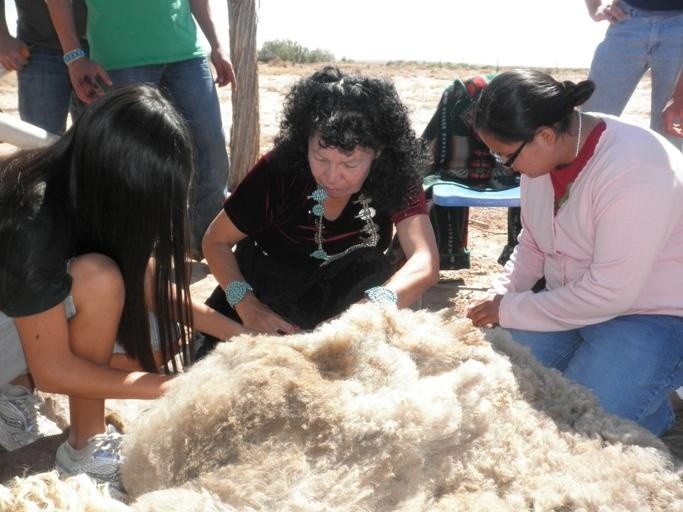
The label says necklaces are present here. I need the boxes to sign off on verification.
[306,186,380,267]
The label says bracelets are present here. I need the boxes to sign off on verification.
[64,49,86,66]
[364,286,398,304]
[225,281,254,309]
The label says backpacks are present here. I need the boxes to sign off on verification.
[432,75,520,193]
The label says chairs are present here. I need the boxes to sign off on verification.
[433,72,529,275]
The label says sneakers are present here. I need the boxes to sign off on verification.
[54,428,128,484]
[0,380,40,454]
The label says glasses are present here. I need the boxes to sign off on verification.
[490,142,527,169]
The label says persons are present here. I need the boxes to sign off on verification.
[0,84,260,483]
[201,68,440,335]
[44,1,236,242]
[579,0,683,152]
[466,68,682,440]
[1,1,89,135]
